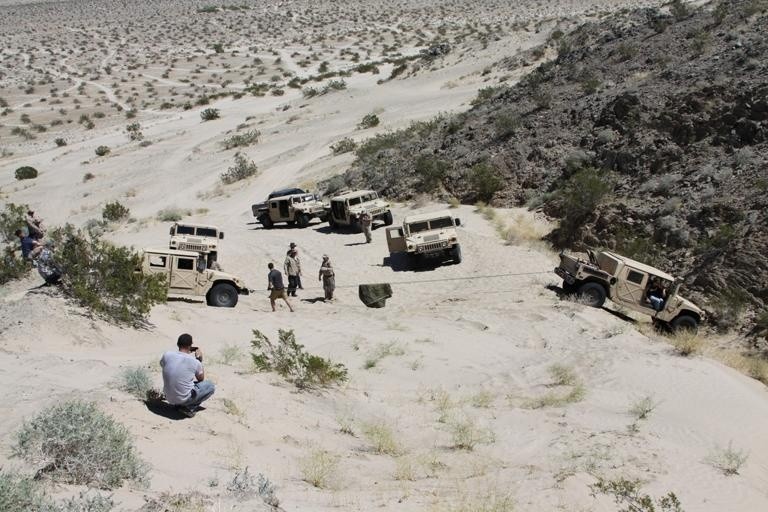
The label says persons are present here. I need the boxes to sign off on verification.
[646,278,668,311]
[208,256,221,270]
[160,334,215,417]
[319,254,335,300]
[284,243,303,297]
[268,263,294,312]
[15,210,62,285]
[359,210,372,243]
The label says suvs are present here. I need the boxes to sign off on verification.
[554,246,705,336]
[386,210,462,269]
[126,222,249,307]
[251,188,393,233]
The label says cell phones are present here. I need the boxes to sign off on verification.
[190,347,198,351]
